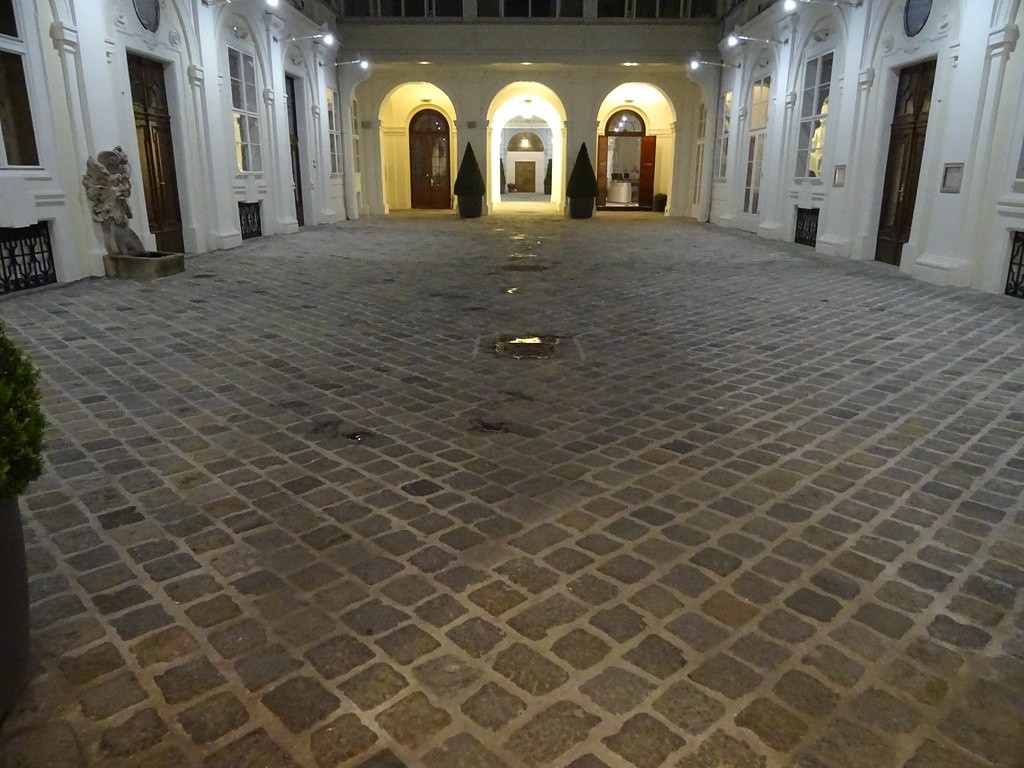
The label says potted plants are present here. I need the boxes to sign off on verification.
[566,142,598,218]
[454,141,486,219]
[654,193,667,212]
[507,183,518,192]
[0,318,48,723]
[545,158,552,194]
[500,158,506,194]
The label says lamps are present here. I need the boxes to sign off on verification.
[690,60,741,70]
[728,31,788,47]
[320,59,368,69]
[274,34,334,45]
[783,0,862,11]
[202,0,278,8]
[519,120,531,148]
